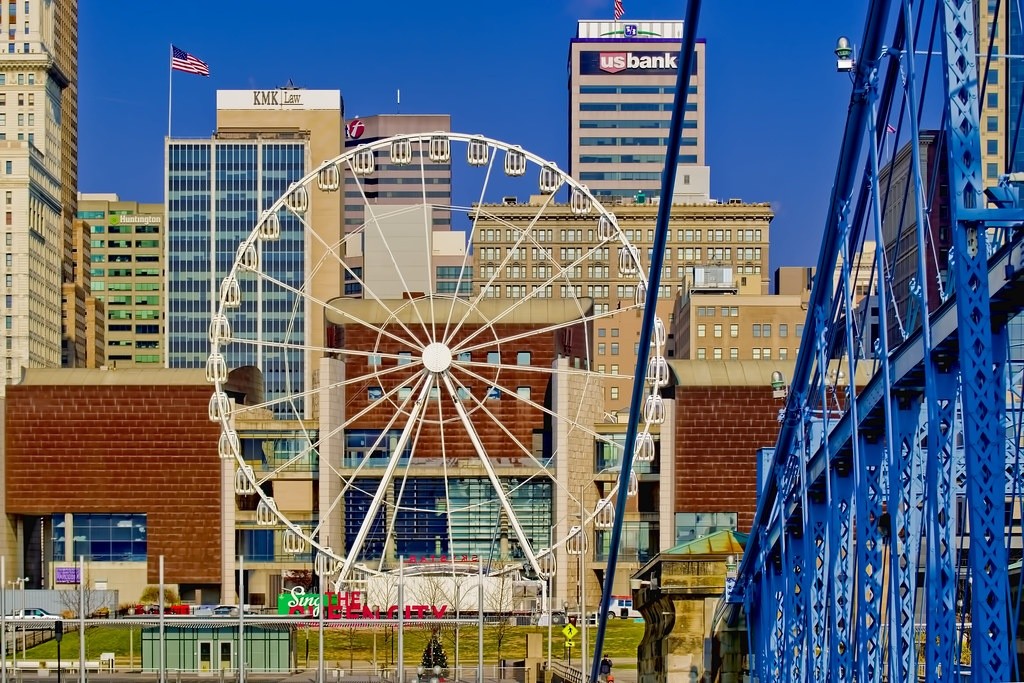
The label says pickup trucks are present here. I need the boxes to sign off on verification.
[0,608,65,632]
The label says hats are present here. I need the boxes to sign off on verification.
[603,653,608,657]
[606,676,614,680]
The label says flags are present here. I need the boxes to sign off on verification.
[171,45,209,78]
[615,0,625,19]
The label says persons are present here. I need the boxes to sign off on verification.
[606,674,615,683]
[599,653,613,681]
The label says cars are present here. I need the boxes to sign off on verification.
[598,600,643,619]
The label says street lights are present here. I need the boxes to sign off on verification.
[8,580,19,677]
[17,577,29,661]
